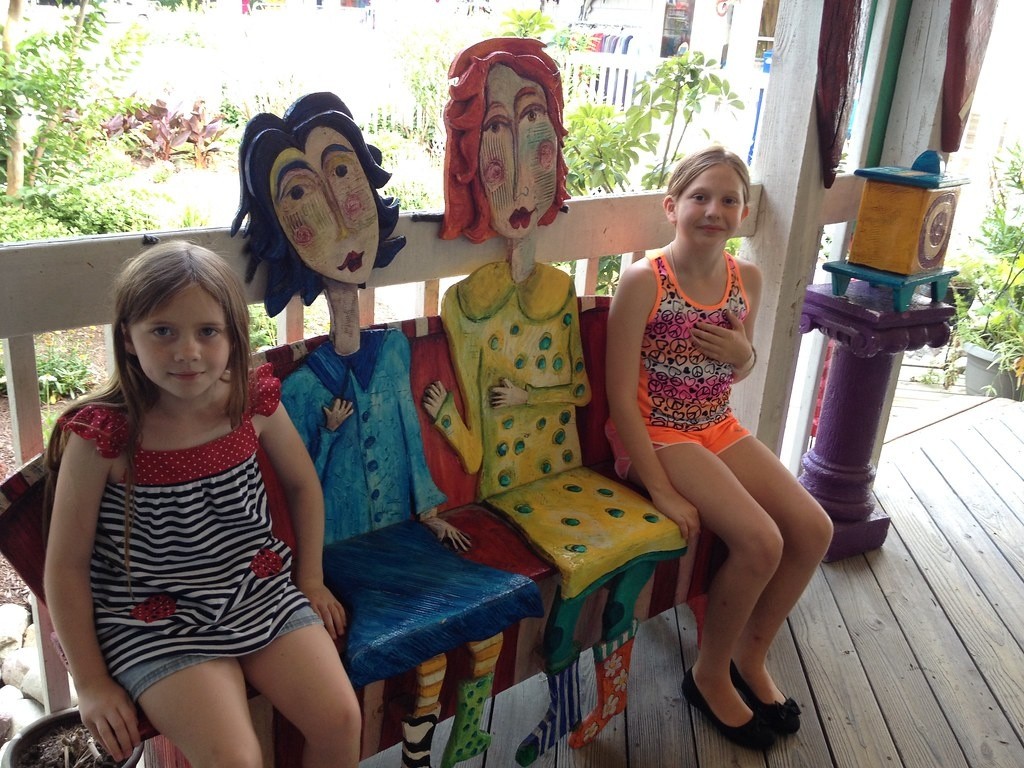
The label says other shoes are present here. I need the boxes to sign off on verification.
[681,664,777,752]
[730,657,802,733]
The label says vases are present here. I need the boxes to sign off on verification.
[1,707,145,768]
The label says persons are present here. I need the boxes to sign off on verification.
[610,148,835,751]
[44,241,362,768]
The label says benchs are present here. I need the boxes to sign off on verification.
[0,293,727,768]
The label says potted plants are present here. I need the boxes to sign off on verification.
[964,308,1024,402]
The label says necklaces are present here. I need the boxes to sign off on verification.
[669,241,731,344]
